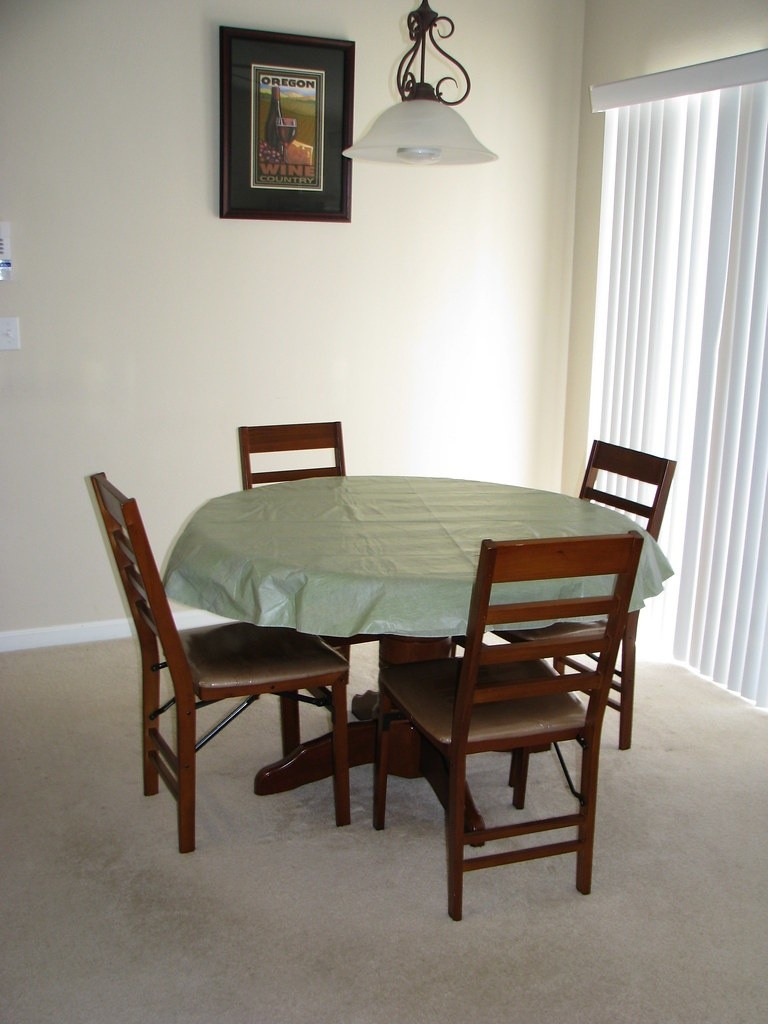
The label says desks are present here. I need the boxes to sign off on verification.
[161,475,676,847]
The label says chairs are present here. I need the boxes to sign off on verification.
[374,530,645,921]
[91,471,350,855]
[238,421,350,660]
[491,439,677,788]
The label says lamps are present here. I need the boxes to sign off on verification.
[342,0,499,166]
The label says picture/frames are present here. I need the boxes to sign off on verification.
[219,26,355,223]
